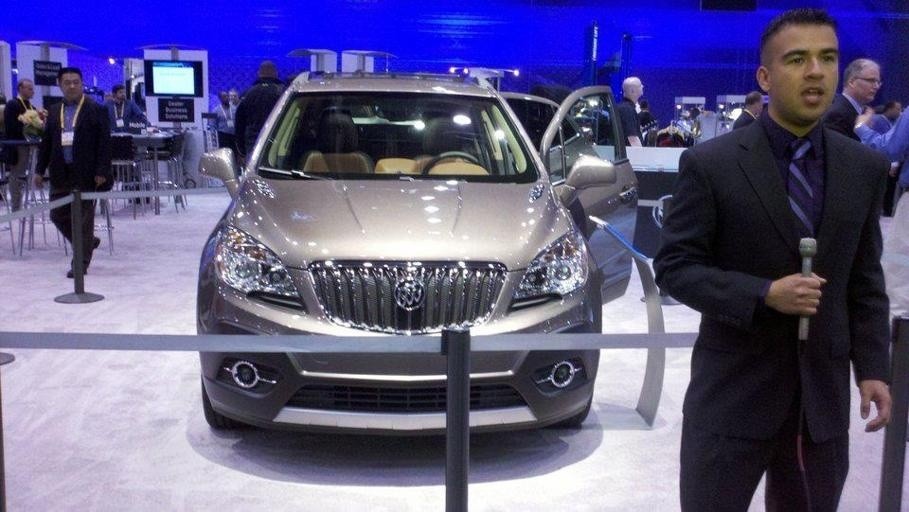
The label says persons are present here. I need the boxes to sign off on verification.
[105,84,152,147]
[654,6,894,510]
[635,99,655,142]
[0,93,8,128]
[611,76,647,150]
[821,55,883,148]
[31,66,114,279]
[235,59,288,177]
[731,91,765,130]
[688,107,716,136]
[228,88,242,109]
[212,90,239,159]
[2,77,44,214]
[850,105,909,223]
[862,99,904,215]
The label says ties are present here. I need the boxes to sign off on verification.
[790,140,816,242]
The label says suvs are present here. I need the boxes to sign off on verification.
[199,62,640,440]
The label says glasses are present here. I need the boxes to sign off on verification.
[856,78,881,85]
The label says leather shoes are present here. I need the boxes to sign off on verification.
[92,237,100,250]
[67,269,87,277]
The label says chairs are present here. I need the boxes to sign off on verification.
[0,124,190,261]
[417,117,478,179]
[301,115,373,178]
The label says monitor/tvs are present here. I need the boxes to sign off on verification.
[143,59,204,97]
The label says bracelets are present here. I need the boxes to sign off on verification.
[853,123,862,131]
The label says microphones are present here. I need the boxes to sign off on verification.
[798,238,818,340]
